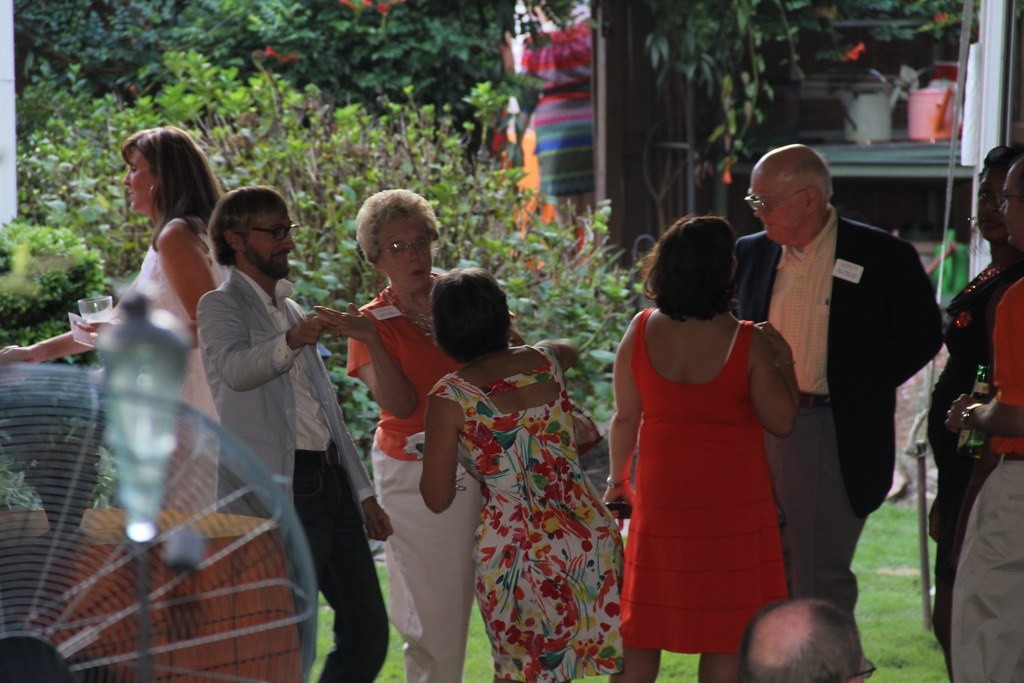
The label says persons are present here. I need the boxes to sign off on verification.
[0,125,225,421]
[419,274,625,683]
[733,596,876,683]
[197,187,389,683]
[501,0,594,229]
[731,142,942,607]
[929,146,1024,683]
[607,214,800,683]
[315,190,526,683]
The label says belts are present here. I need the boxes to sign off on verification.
[295,443,339,469]
[990,452,1024,461]
[800,393,828,409]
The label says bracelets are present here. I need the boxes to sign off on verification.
[774,360,796,369]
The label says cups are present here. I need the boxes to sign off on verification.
[78,296,113,344]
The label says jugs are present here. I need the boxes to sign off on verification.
[845,67,904,143]
[907,66,959,140]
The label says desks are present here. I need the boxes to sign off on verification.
[0,507,304,683]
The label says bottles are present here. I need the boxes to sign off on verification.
[958,365,993,462]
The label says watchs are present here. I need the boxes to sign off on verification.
[606,475,629,488]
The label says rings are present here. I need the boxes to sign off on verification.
[336,333,340,337]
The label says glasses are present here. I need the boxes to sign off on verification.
[379,236,431,256]
[997,193,1024,204]
[849,658,877,679]
[744,186,813,210]
[250,225,300,240]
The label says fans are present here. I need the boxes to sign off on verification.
[0,359,320,683]
